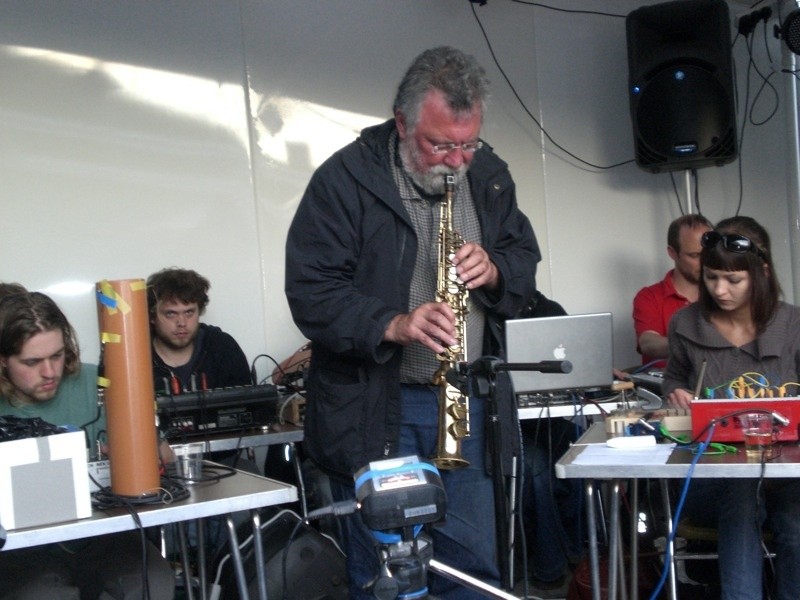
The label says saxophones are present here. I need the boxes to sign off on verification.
[430,172,473,470]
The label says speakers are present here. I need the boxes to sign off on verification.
[625,1,740,172]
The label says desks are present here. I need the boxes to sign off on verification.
[516,371,800,600]
[0,458,299,600]
[163,417,310,573]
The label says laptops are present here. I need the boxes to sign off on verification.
[503,313,613,392]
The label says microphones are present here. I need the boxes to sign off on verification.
[445,368,469,395]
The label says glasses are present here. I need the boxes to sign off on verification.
[700,231,768,263]
[409,113,484,155]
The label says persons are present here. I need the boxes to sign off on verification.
[286,46,541,600]
[97,269,251,584]
[633,214,714,368]
[273,342,312,386]
[495,291,582,600]
[0,283,176,600]
[662,216,800,600]
